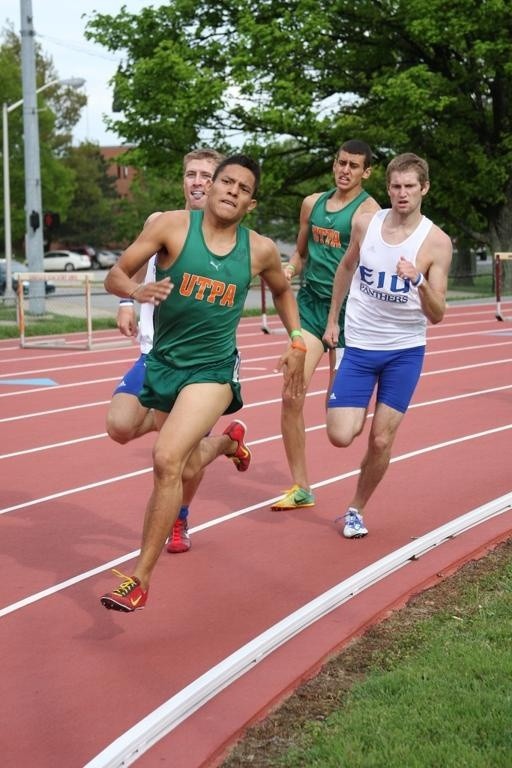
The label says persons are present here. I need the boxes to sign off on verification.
[100,154,311,613]
[106,148,224,553]
[270,140,381,510]
[322,152,454,539]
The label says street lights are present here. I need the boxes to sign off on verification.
[2,76,85,304]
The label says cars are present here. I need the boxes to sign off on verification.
[0,259,54,295]
[24,244,124,274]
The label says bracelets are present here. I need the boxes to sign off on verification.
[130,283,146,298]
[290,330,304,339]
[286,263,297,276]
[413,272,423,287]
[118,300,135,307]
[292,342,307,353]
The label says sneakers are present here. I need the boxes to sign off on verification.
[271,486,314,511]
[343,507,368,537]
[223,420,250,471]
[103,576,148,612]
[167,520,190,553]
